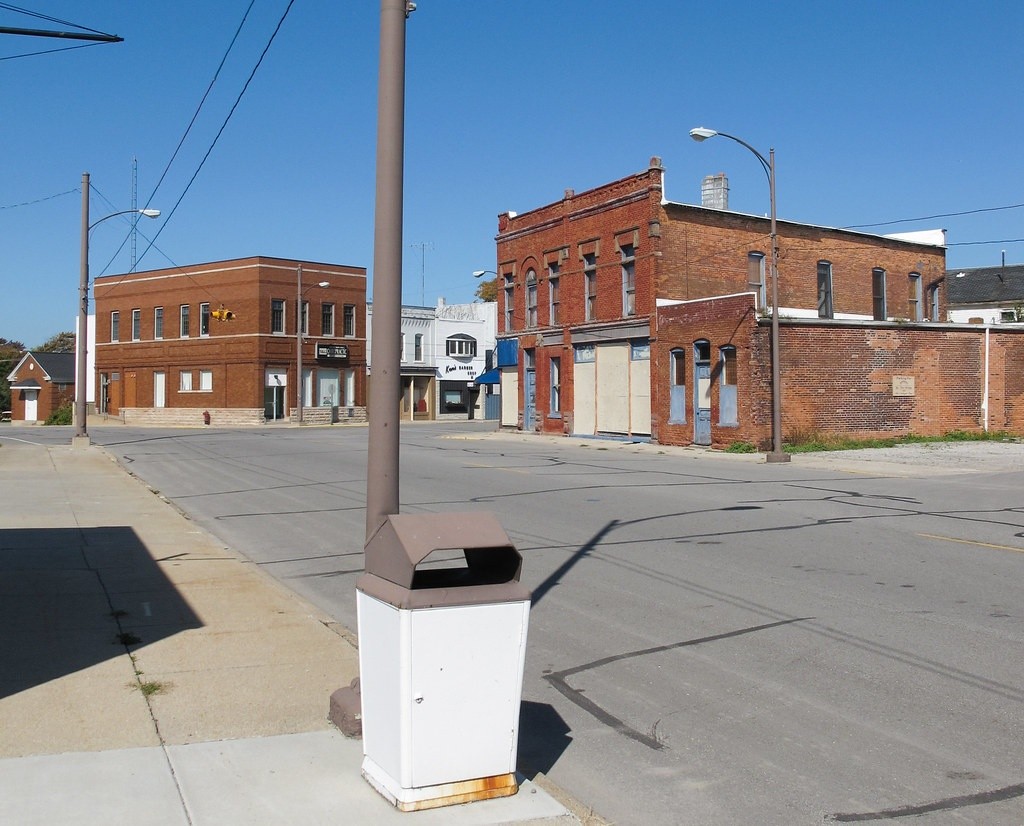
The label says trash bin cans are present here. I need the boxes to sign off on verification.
[352,507,533,815]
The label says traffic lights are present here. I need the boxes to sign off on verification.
[224,310,233,322]
[211,306,221,321]
[232,313,238,320]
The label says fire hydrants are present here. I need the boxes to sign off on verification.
[202,410,214,425]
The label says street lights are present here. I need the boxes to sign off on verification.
[687,125,799,463]
[70,208,159,445]
[295,264,330,419]
